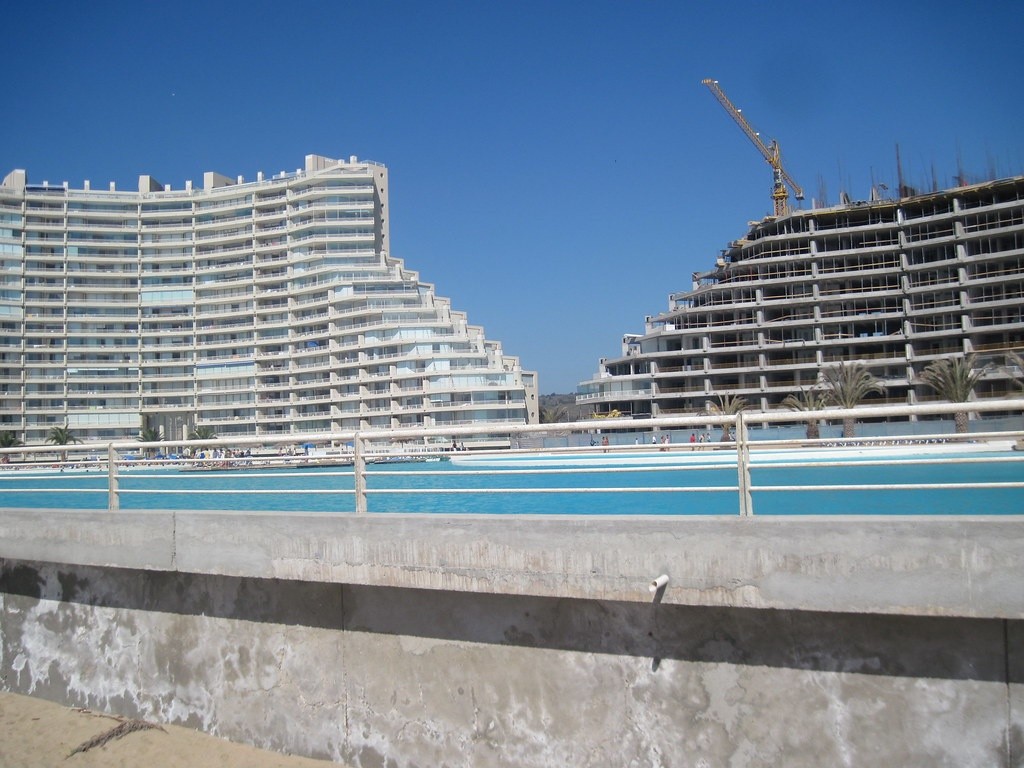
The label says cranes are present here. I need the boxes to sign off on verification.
[700,77,806,217]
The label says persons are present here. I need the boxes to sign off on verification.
[277,447,292,456]
[304,445,309,454]
[96,455,102,468]
[181,446,253,467]
[453,441,465,451]
[727,430,737,441]
[689,433,713,452]
[601,436,609,453]
[651,433,670,453]
[635,438,639,445]
